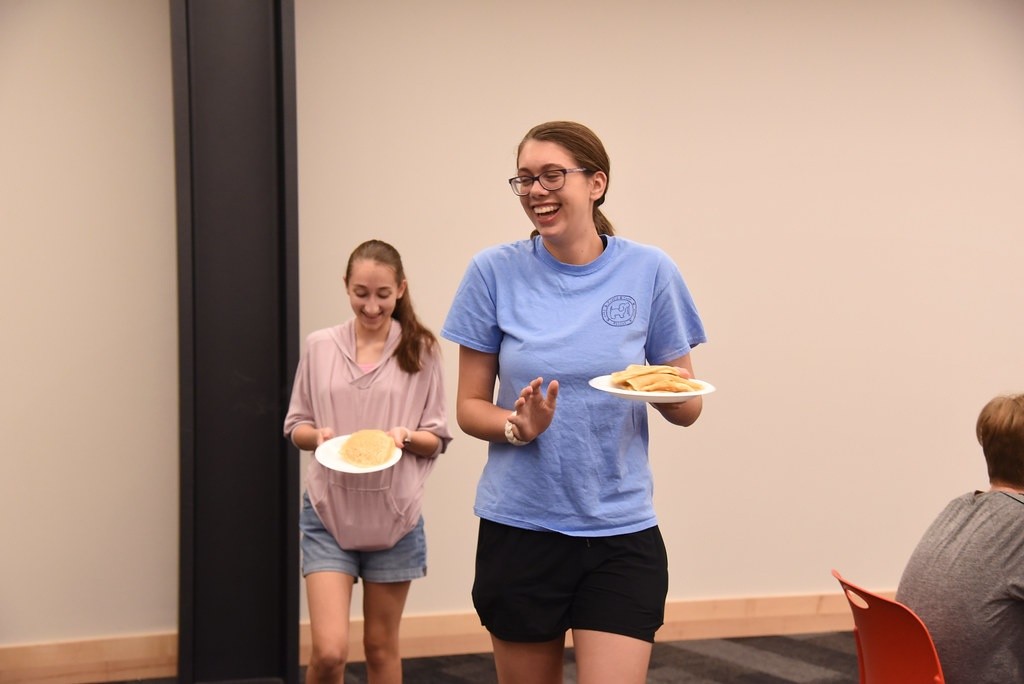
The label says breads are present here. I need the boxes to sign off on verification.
[341,429,395,468]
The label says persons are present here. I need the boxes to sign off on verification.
[895,394,1024,684]
[284,239,453,684]
[441,121,707,684]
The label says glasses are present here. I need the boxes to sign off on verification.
[508,167,588,197]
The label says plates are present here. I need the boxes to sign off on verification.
[588,375,714,403]
[314,435,403,473]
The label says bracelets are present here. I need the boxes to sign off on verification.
[402,427,411,447]
[505,411,532,446]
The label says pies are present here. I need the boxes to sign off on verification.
[609,364,706,393]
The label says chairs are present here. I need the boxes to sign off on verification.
[831,567,946,684]
